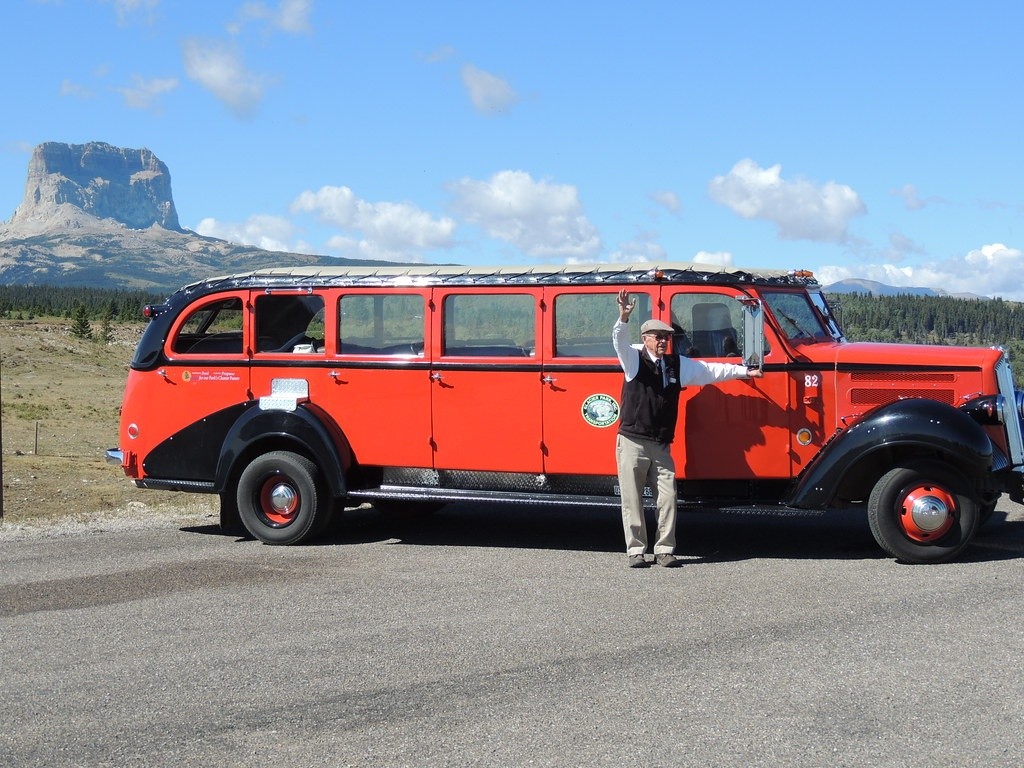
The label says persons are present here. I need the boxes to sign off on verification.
[611,288,764,569]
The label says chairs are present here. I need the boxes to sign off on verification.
[645,308,693,356]
[691,301,740,357]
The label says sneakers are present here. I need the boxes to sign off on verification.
[628,555,646,568]
[654,552,679,568]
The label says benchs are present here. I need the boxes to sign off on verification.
[337,335,618,360]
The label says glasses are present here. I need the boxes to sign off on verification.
[645,332,671,341]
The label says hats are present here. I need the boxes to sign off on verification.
[640,319,675,334]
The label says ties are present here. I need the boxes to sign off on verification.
[656,358,664,391]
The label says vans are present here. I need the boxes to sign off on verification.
[104,257,1024,564]
